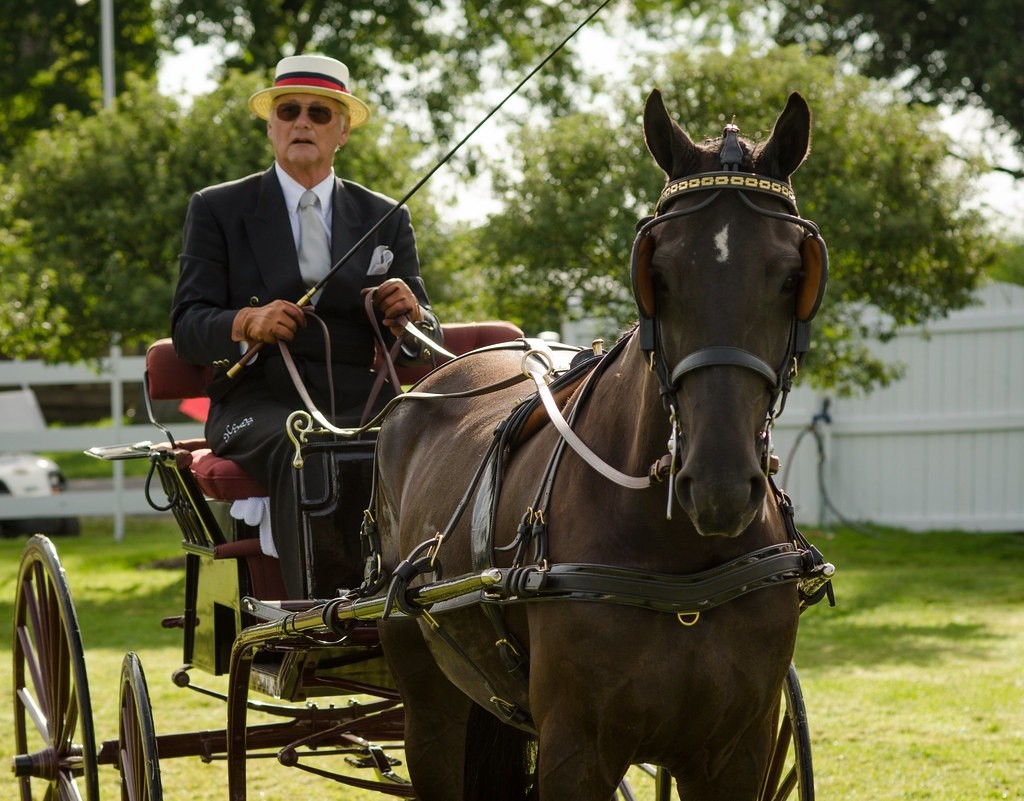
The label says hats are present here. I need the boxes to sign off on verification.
[248,55,370,130]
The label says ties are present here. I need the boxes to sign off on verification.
[296,190,332,308]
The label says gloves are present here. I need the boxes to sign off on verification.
[360,276,426,340]
[230,300,306,345]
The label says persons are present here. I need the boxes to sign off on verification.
[168,54,441,612]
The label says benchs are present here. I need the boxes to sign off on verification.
[143,318,524,594]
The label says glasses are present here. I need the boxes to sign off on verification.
[271,102,345,124]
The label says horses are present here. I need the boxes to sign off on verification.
[369,83,815,801]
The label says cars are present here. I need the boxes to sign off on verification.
[0,387,82,538]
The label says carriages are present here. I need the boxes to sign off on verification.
[11,89,837,801]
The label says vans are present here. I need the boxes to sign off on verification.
[0,356,213,466]
